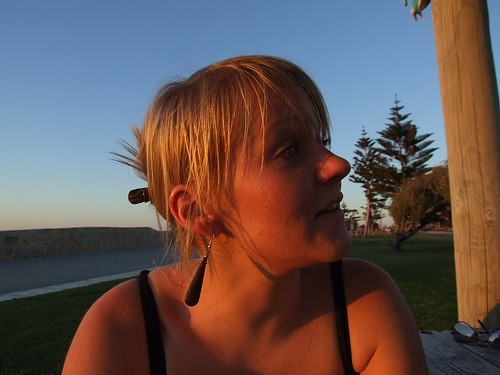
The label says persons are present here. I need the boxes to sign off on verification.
[62,55,431,374]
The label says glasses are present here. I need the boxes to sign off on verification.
[449,317,500,350]
